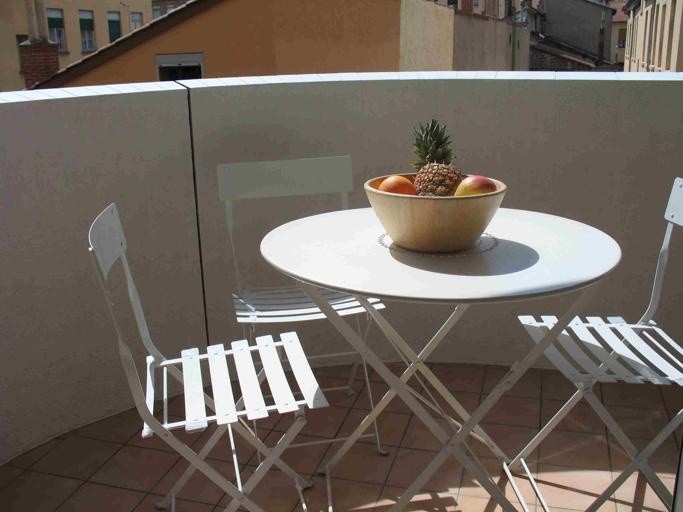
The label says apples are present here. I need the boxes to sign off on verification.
[454,175,496,196]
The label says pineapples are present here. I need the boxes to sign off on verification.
[414,163,461,197]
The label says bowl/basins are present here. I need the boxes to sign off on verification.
[364,171,508,255]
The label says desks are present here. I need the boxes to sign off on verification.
[257,196,624,512]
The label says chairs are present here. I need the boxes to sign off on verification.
[86,202,334,511]
[501,169,683,512]
[212,153,390,473]
